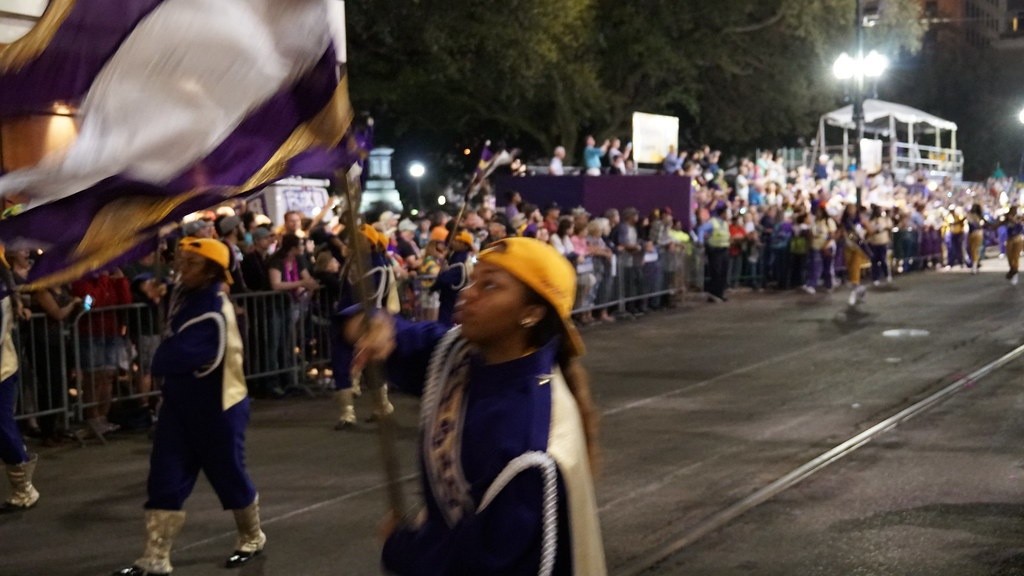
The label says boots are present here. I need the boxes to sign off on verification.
[349,359,362,396]
[231,493,266,560]
[6,453,39,507]
[122,508,186,576]
[334,389,356,423]
[372,384,394,418]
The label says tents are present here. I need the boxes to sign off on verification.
[816,99,958,171]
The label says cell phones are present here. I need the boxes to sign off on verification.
[84,295,93,311]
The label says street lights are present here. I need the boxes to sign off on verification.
[404,157,427,211]
[830,1,883,222]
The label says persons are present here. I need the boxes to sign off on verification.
[0,136,1023,508]
[116,237,266,576]
[332,239,609,575]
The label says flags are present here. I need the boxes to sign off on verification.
[0,0,366,289]
[465,143,514,202]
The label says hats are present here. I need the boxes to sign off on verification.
[477,235,585,353]
[380,211,401,224]
[181,238,235,284]
[431,227,449,241]
[455,228,477,251]
[360,224,379,244]
[399,219,417,230]
[379,231,390,250]
[485,211,514,231]
